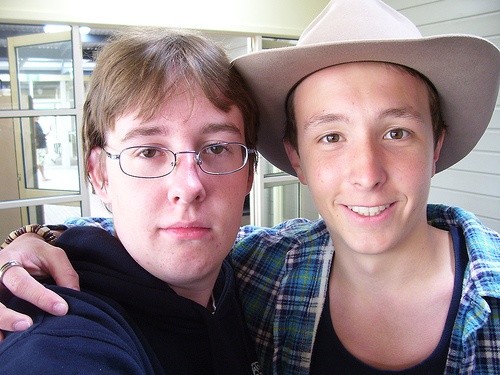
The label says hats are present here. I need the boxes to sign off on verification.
[231,0,500,177]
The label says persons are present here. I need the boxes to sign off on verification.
[0,30,266,375]
[0,0,500,375]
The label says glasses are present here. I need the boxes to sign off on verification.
[102,143,256,178]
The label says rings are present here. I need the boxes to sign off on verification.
[0,260,23,282]
